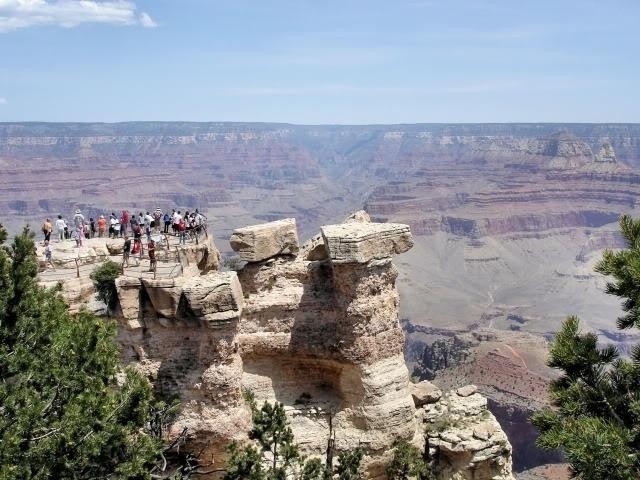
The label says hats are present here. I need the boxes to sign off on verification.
[75,209,81,213]
[100,216,104,218]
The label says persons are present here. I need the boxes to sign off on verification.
[40,206,208,272]
